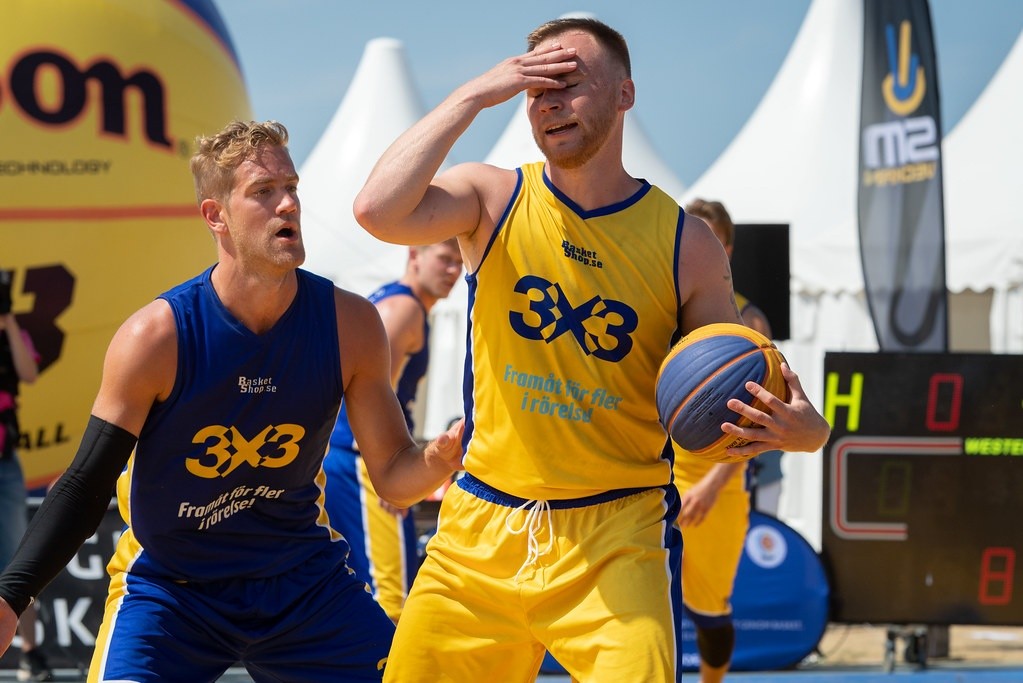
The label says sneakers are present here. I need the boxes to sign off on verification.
[17,648,55,682]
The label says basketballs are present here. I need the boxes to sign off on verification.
[655,322,792,465]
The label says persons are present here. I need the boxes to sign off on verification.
[352,16,834,683]
[671,198,774,683]
[314,235,464,626]
[0,119,469,683]
[0,288,64,683]
